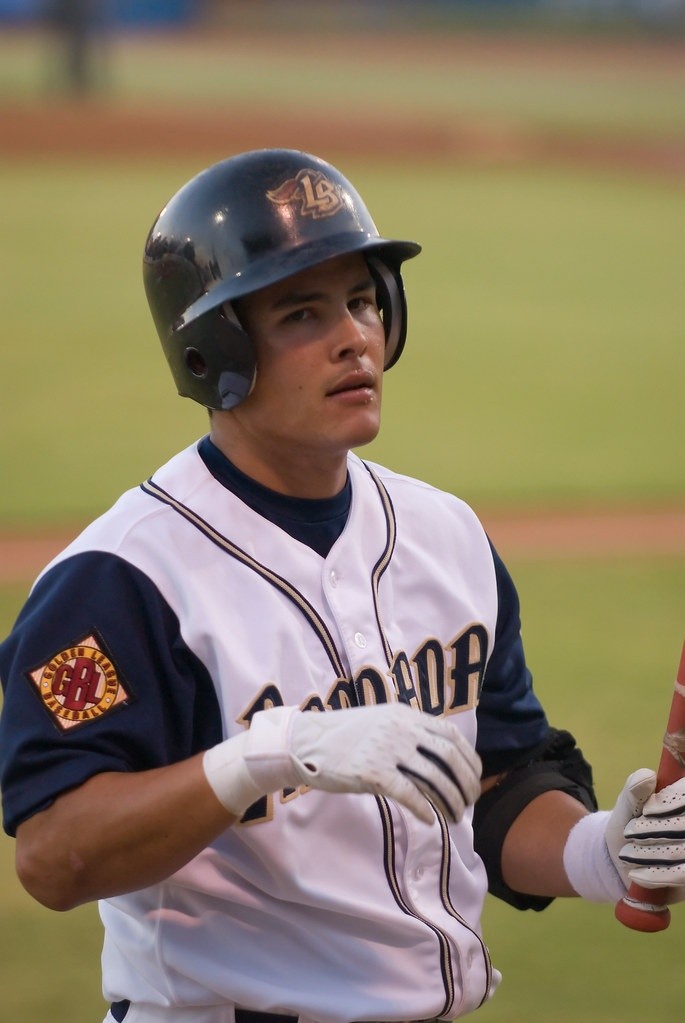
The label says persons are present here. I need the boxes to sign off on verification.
[1,147,685,1023]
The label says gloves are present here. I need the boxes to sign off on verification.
[604,768,685,905]
[244,704,484,825]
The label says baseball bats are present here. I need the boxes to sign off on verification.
[617,638,685,932]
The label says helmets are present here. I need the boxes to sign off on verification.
[144,145,421,413]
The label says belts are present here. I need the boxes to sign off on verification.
[111,999,299,1023]
